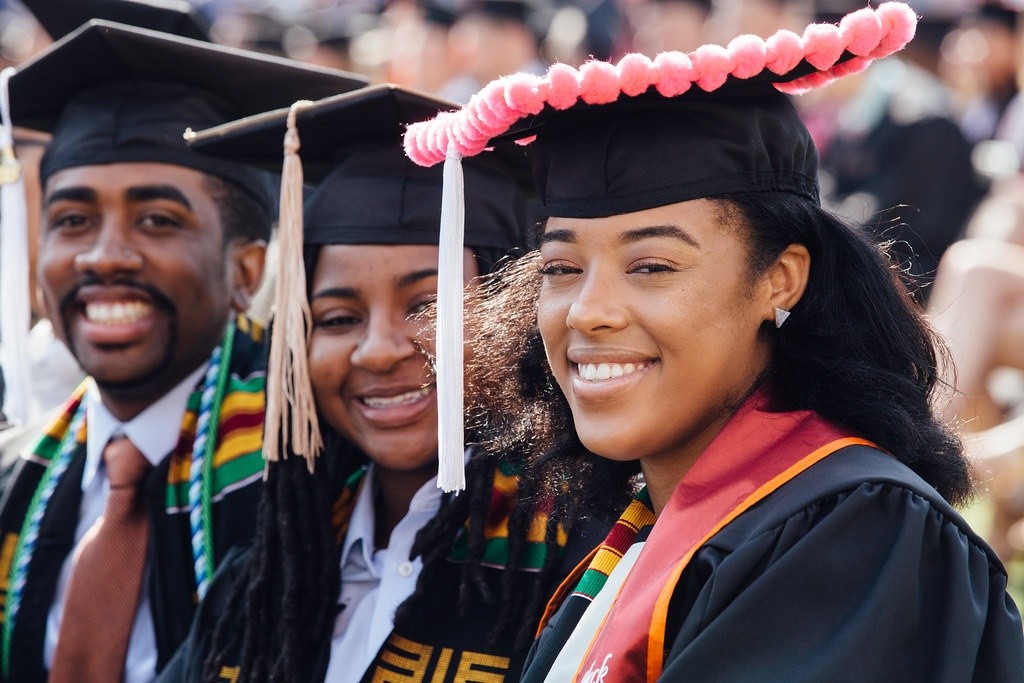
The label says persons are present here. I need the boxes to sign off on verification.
[0,22,371,683]
[147,83,639,682]
[0,0,1024,567]
[395,1,1024,683]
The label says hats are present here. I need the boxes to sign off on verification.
[0,17,372,427]
[403,2,917,496]
[183,83,524,481]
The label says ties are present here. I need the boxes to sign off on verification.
[47,437,151,683]
